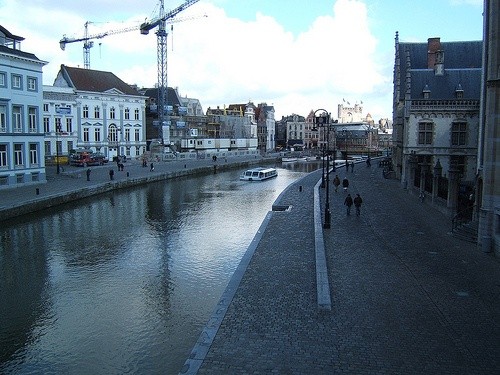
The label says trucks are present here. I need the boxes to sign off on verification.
[69,152,109,167]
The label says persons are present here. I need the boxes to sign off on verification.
[117,155,154,173]
[333,156,372,192]
[110,168,114,181]
[87,169,91,181]
[211,155,217,162]
[354,194,362,216]
[344,194,353,217]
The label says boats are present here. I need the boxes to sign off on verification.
[320,156,331,161]
[239,167,278,182]
[281,156,317,162]
[329,155,368,165]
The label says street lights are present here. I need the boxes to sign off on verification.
[311,108,332,229]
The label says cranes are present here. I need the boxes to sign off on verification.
[59,13,208,69]
[139,0,202,142]
